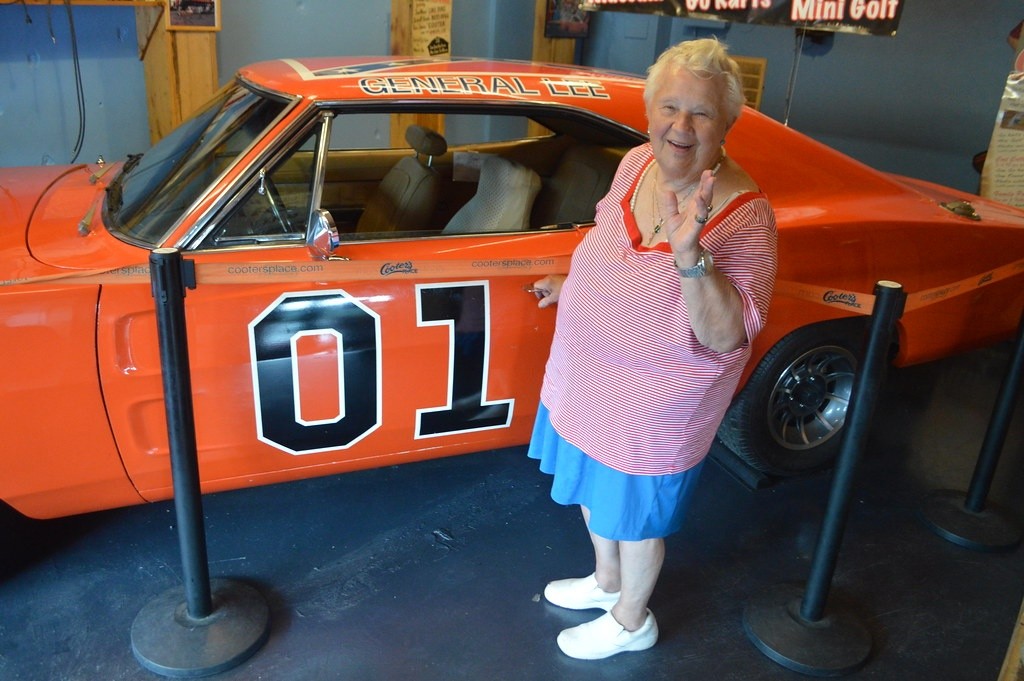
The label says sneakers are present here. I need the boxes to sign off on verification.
[544,570,623,612]
[557,609,658,659]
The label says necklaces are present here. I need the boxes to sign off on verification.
[646,157,728,246]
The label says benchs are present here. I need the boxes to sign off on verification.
[534,142,629,227]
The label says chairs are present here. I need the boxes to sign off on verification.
[355,121,447,232]
[441,157,542,234]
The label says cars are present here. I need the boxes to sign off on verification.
[1,57,1024,521]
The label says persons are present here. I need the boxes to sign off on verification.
[530,36,780,661]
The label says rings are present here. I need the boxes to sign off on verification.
[694,213,708,223]
[706,204,713,213]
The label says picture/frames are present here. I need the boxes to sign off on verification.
[165,0,222,31]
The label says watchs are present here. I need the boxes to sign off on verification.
[672,246,714,277]
